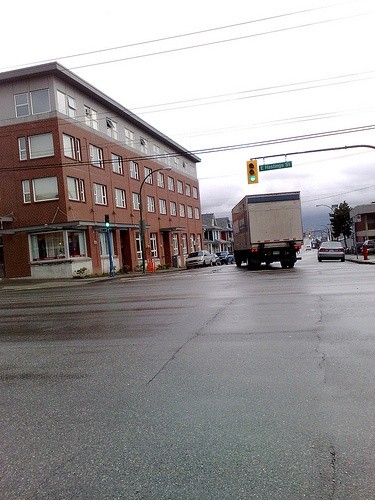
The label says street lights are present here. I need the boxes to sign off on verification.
[316,204,339,241]
[321,224,330,240]
[138,166,172,274]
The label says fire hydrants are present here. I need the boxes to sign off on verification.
[361,244,369,260]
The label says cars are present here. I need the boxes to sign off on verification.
[306,245,311,251]
[360,239,375,255]
[216,251,235,265]
[317,241,346,262]
[185,250,214,268]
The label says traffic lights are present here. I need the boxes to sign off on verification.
[308,232,311,237]
[104,214,110,228]
[246,159,259,184]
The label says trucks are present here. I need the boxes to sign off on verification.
[231,190,305,270]
